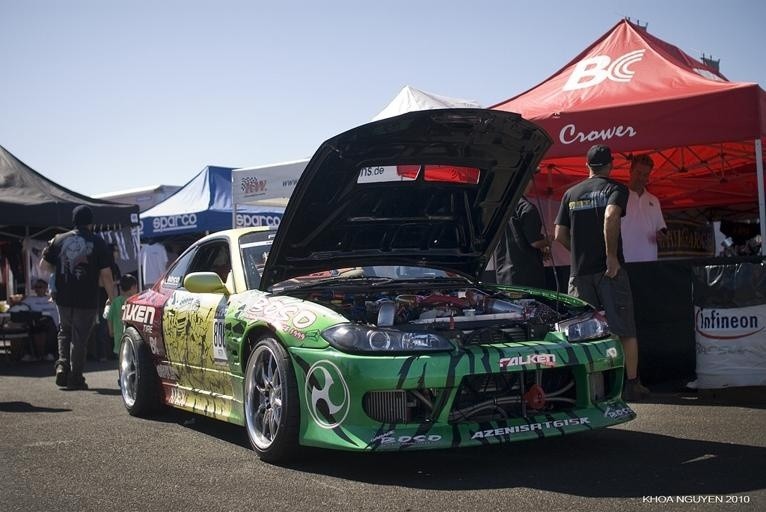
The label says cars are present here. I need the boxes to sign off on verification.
[118,108,637,463]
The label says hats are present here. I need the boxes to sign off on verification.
[586,144,615,166]
[72,205,97,218]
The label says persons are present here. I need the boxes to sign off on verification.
[107,273,145,386]
[23,280,61,362]
[555,144,651,395]
[620,152,670,396]
[37,204,115,390]
[493,170,550,289]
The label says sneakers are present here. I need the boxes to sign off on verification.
[621,379,649,402]
[55,363,69,386]
[67,375,88,390]
